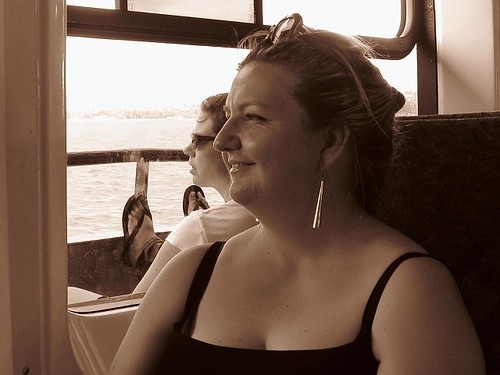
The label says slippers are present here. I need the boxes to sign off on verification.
[182,185,210,217]
[122,195,152,267]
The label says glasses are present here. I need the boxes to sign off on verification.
[191,130,215,149]
[264,12,370,114]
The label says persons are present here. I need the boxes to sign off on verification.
[129,93,255,299]
[116,183,215,274]
[103,12,490,374]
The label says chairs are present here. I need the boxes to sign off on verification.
[370,111,500,375]
